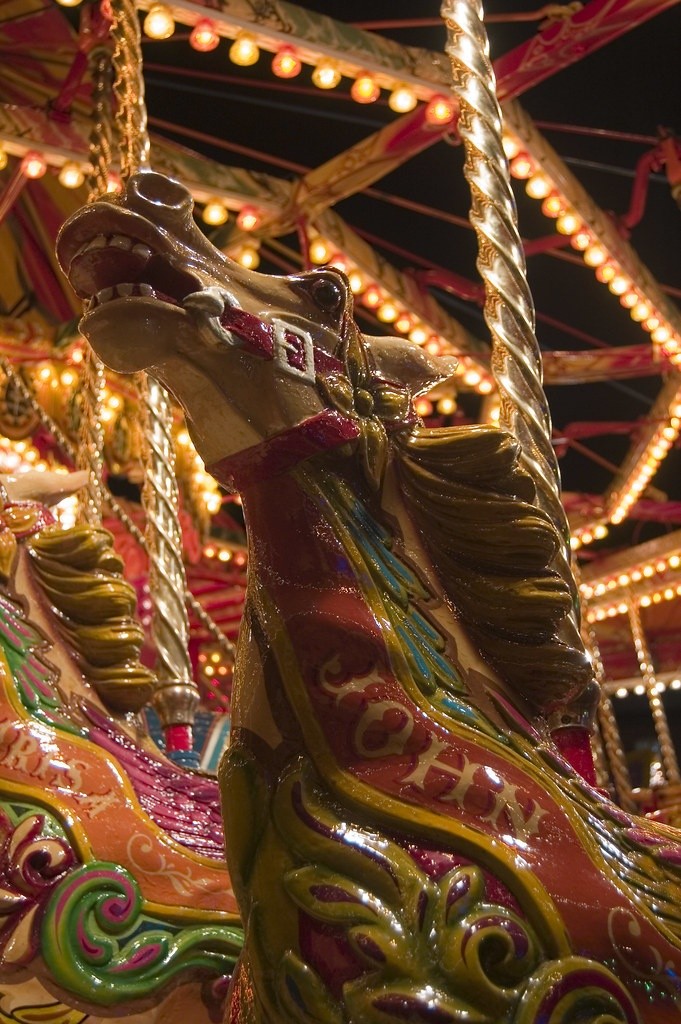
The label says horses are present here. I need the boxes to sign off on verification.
[0,481,245,1024]
[54,172,679,1024]
